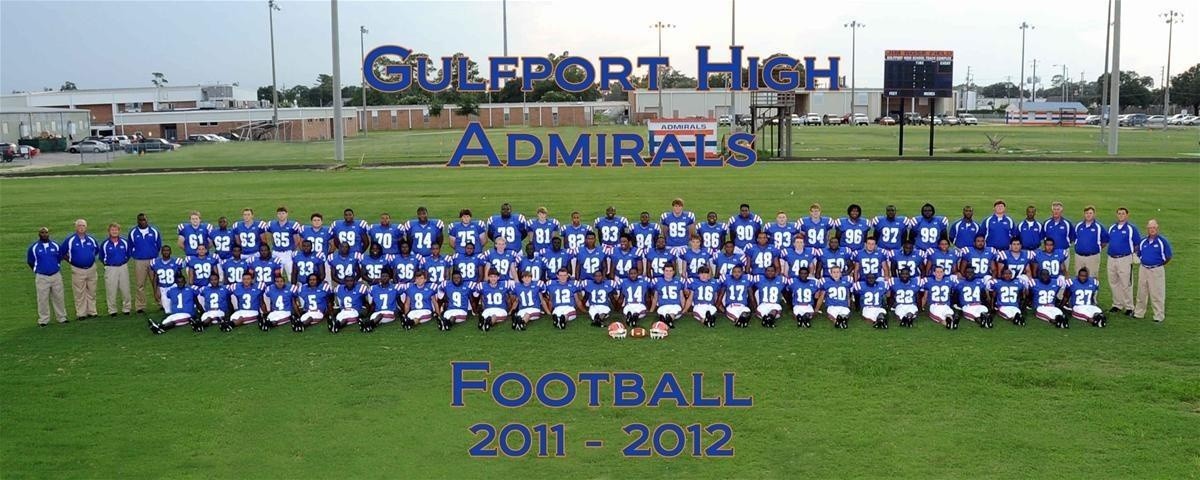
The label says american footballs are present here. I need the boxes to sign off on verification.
[629,328,650,337]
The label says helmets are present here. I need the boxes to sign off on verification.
[651,321,669,340]
[608,321,626,339]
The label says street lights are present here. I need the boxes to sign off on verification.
[269,0,283,126]
[845,20,866,122]
[1158,11,1185,128]
[360,25,369,139]
[151,73,168,111]
[1020,21,1036,132]
[1053,64,1067,102]
[649,21,675,119]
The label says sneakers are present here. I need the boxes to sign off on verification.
[401,314,566,331]
[872,313,1107,329]
[1124,310,1134,316]
[147,315,375,334]
[1110,306,1121,312]
[595,311,848,331]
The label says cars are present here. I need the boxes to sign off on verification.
[1085,114,1200,128]
[720,112,977,126]
[67,132,249,153]
[18,144,40,160]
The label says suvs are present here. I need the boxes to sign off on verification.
[0,143,17,163]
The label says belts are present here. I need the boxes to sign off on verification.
[1111,255,1127,258]
[1142,264,1161,268]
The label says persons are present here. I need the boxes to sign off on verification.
[99,223,132,316]
[136,132,146,158]
[127,213,164,313]
[61,219,99,320]
[27,227,70,328]
[149,197,1172,335]
